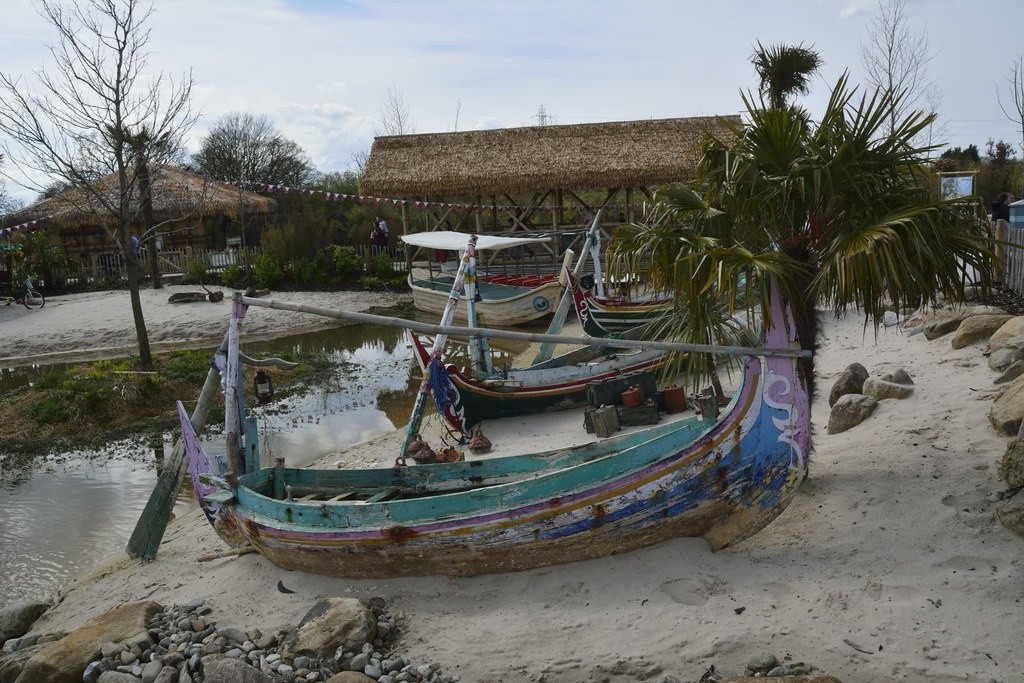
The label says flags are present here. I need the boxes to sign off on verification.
[208,181,641,213]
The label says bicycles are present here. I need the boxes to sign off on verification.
[0,270,46,311]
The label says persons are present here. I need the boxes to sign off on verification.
[371,217,388,252]
[130,231,148,258]
[992,192,1014,222]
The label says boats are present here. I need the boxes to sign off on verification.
[561,245,763,341]
[177,263,816,576]
[399,228,575,319]
[403,301,764,434]
